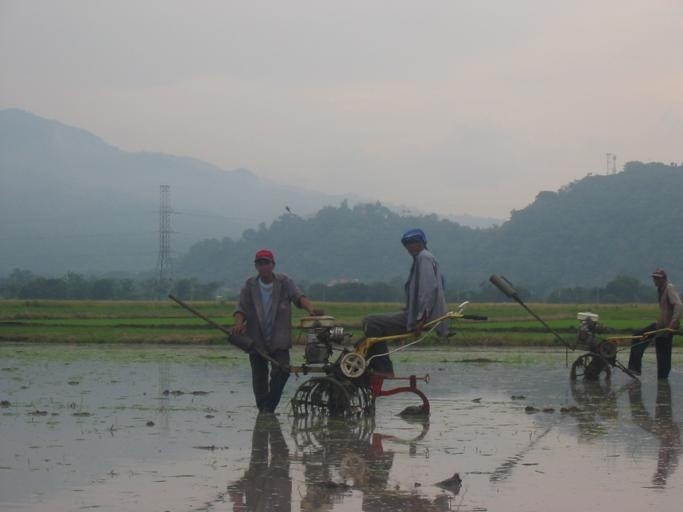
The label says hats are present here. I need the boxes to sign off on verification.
[401,228,427,245]
[255,250,275,263]
[651,268,666,278]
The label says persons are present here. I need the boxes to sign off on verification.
[228,249,323,417]
[622,377,682,490]
[360,228,449,380]
[223,412,296,511]
[623,268,681,380]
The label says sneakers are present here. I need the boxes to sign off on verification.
[370,367,394,379]
